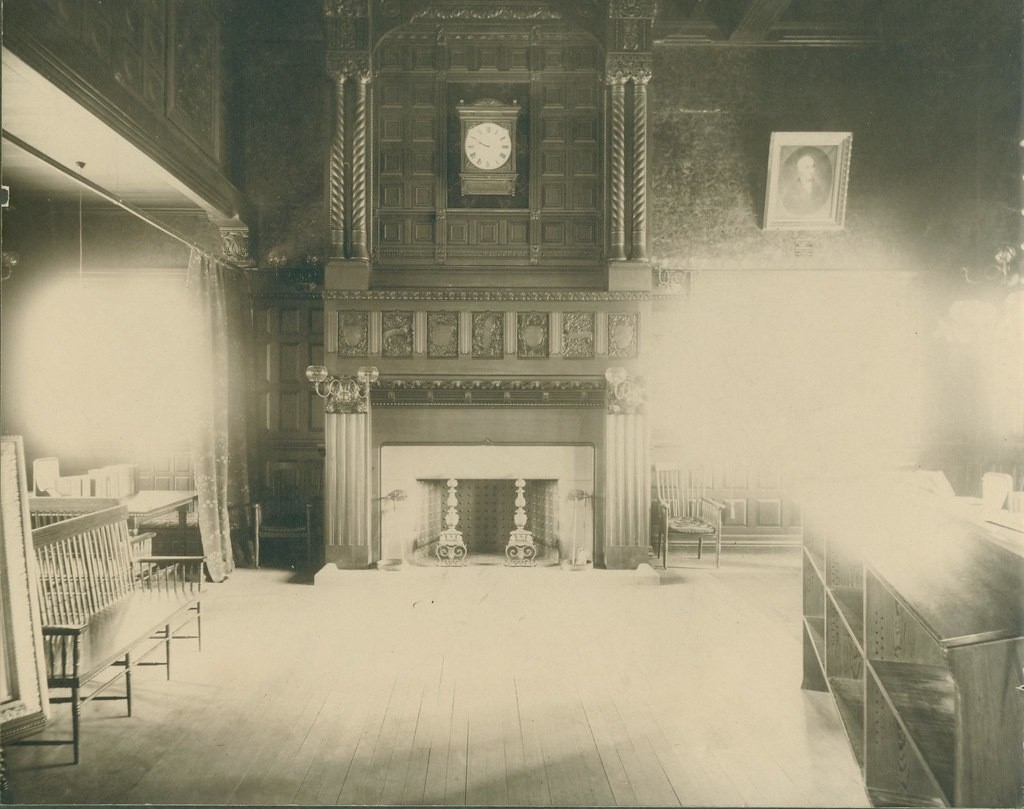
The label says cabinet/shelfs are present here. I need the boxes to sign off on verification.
[798,497,1023,808]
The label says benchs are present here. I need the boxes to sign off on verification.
[28,495,208,765]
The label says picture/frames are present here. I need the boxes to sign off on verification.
[761,131,852,230]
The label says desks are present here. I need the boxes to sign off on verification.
[26,488,199,556]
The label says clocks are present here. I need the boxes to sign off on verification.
[455,98,522,195]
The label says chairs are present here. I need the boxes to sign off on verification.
[253,452,317,568]
[656,462,726,570]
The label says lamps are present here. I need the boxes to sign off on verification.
[606,367,645,401]
[0,250,19,282]
[305,365,379,407]
